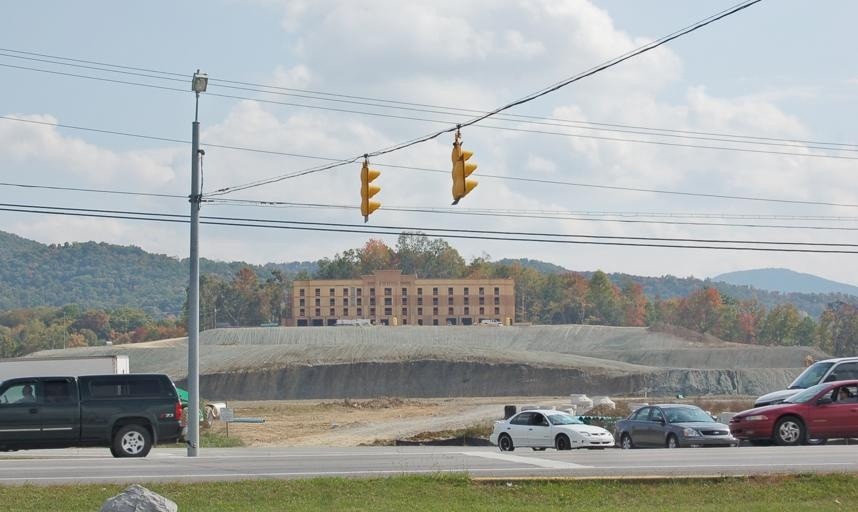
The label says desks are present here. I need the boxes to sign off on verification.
[189,71,207,454]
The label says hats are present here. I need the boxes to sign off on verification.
[489,410,614,451]
[616,405,733,448]
[730,379,858,445]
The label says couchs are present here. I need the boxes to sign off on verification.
[361,166,380,221]
[450,144,477,205]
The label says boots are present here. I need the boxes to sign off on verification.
[755,355,858,406]
[1,376,184,458]
